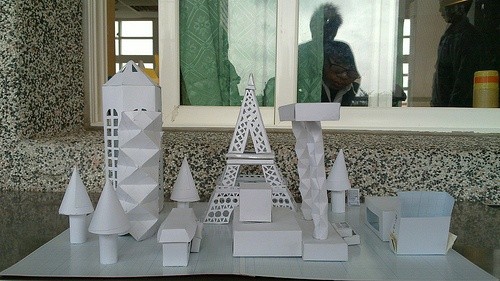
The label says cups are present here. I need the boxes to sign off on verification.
[473,70,499,108]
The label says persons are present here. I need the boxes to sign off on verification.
[293,1,359,106]
[430,0,486,108]
[297,47,360,105]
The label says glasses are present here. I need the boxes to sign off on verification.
[327,57,359,78]
